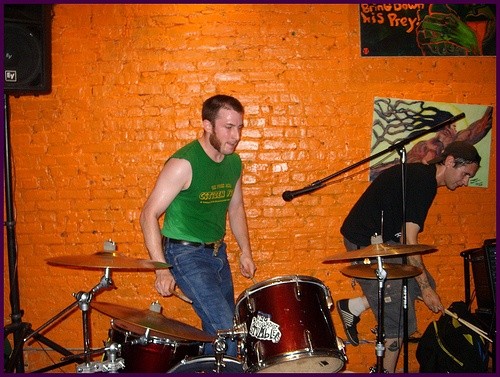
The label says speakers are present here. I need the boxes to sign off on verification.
[4,5,53,97]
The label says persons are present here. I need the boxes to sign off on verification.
[337,142,481,374]
[139,95,256,357]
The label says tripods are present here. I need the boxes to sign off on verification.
[3,95,86,373]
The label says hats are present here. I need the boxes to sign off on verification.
[428,140,479,165]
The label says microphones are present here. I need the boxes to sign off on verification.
[282,184,327,202]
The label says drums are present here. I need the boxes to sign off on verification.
[103,318,206,373]
[163,354,250,373]
[234,275,346,373]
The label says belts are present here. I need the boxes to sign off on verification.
[162,236,215,250]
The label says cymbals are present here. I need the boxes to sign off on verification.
[43,253,176,268]
[89,302,218,342]
[342,262,424,279]
[319,245,438,261]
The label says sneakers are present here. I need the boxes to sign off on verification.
[337,299,360,346]
